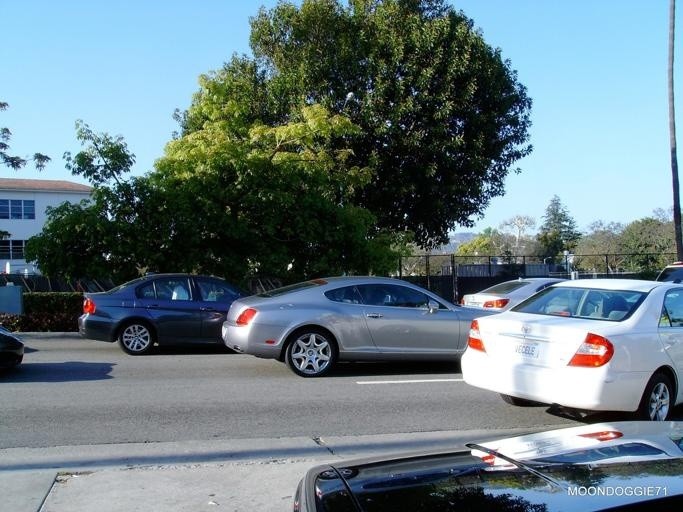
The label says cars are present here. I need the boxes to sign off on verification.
[456,260,683,422]
[286,415,682,512]
[216,274,505,380]
[77,271,253,357]
[464,420,683,475]
[0,324,28,377]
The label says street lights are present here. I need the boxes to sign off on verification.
[562,250,569,279]
